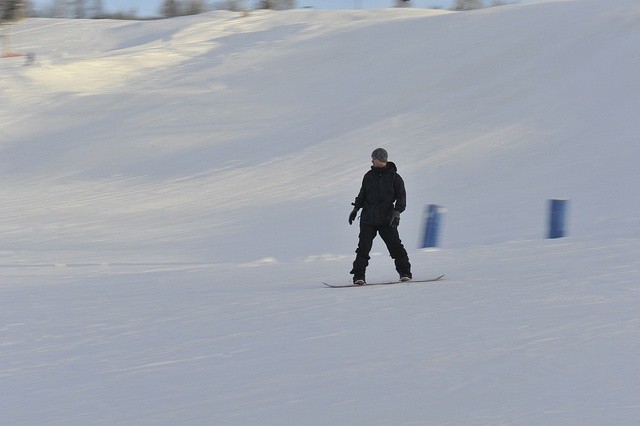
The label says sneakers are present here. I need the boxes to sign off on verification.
[353,274,365,283]
[400,272,412,281]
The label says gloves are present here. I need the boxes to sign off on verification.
[348,210,357,225]
[389,211,400,228]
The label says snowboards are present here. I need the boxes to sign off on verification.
[323,274,446,288]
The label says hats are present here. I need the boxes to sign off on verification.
[371,148,388,163]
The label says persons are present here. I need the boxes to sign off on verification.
[348,148,412,284]
